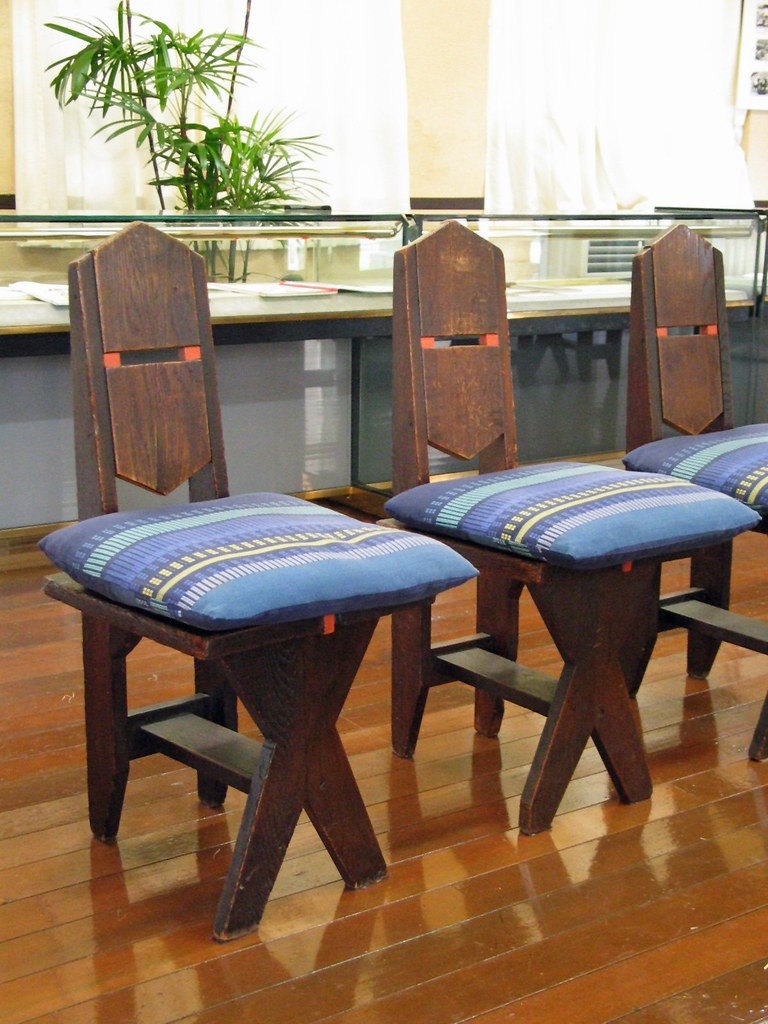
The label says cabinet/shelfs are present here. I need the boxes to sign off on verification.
[1,206,768,361]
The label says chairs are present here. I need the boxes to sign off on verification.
[376,221,721,834]
[619,223,768,762]
[39,220,436,940]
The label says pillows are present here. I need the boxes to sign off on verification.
[623,423,768,521]
[385,463,762,569]
[40,490,482,628]
